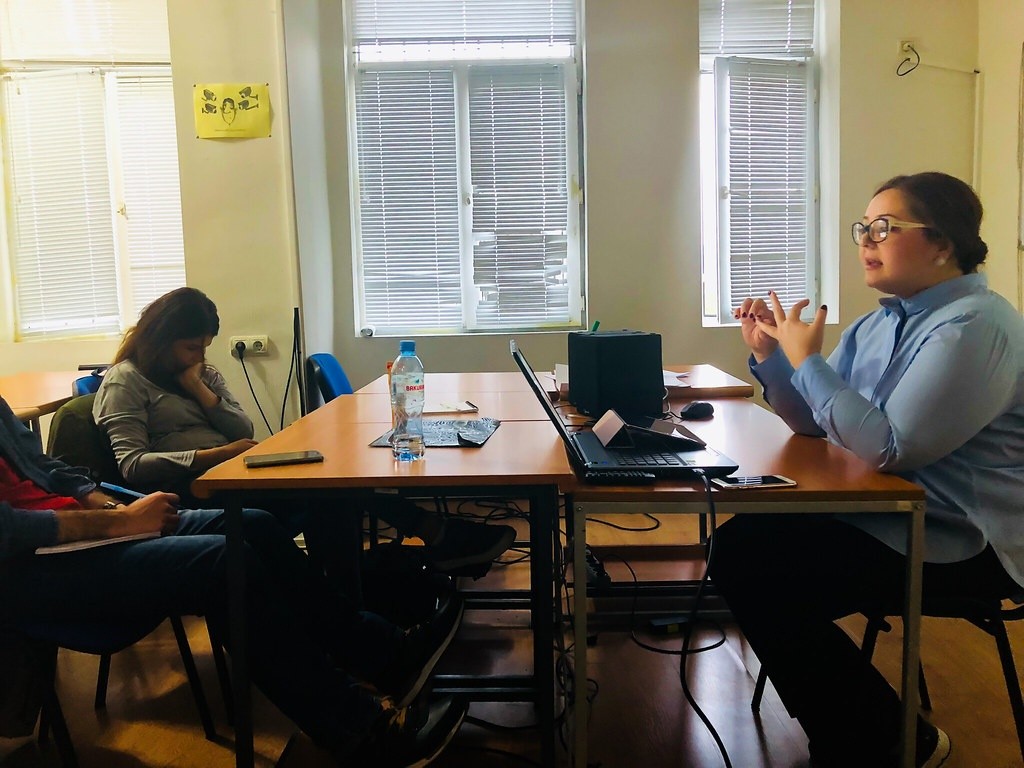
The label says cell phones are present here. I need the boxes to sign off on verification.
[711,475,797,489]
[243,450,324,467]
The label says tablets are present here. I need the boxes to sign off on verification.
[611,407,707,447]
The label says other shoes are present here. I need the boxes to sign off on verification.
[807,698,951,768]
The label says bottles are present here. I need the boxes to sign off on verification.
[390,340,426,462]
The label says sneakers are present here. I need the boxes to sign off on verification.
[389,595,464,708]
[337,690,469,768]
[429,522,516,572]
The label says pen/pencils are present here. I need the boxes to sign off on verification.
[101,482,185,509]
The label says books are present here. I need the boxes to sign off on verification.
[35,529,161,554]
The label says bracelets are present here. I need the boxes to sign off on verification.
[103,497,127,511]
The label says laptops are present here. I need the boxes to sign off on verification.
[509,339,739,485]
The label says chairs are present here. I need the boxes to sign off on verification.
[46,388,218,736]
[308,352,354,405]
[751,555,1024,768]
[0,569,217,768]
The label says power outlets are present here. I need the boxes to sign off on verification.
[231,335,270,357]
[897,39,915,56]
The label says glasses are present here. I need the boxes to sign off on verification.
[851,218,935,245]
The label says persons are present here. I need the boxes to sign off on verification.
[706,171,1024,768]
[0,397,469,768]
[92,287,517,625]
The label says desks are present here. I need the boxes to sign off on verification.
[188,372,931,768]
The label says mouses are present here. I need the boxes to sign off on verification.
[680,401,714,419]
